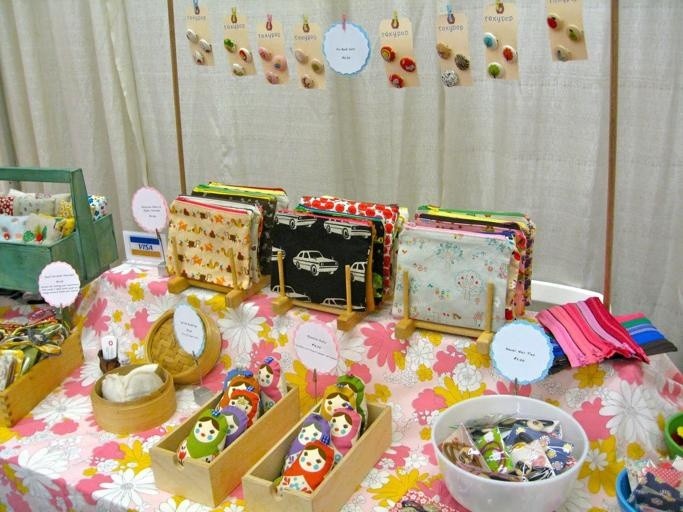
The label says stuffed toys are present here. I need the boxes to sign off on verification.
[272,375,368,497]
[176,356,287,467]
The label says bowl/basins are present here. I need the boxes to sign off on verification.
[614,458,660,512]
[664,411,682,456]
[428,395,592,512]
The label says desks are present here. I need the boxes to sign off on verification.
[1,254,683,512]
